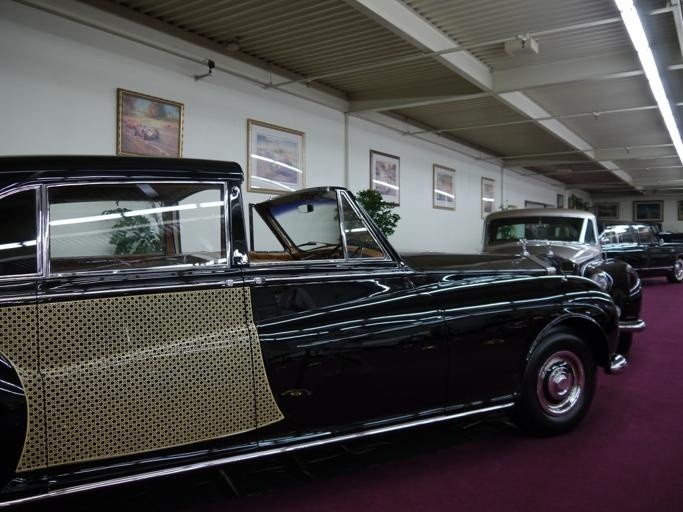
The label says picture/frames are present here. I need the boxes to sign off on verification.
[432,163,457,211]
[369,148,400,208]
[115,87,185,160]
[246,116,307,195]
[480,176,495,219]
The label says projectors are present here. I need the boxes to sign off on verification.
[504,38,538,58]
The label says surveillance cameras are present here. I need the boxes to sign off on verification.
[205,58,215,68]
